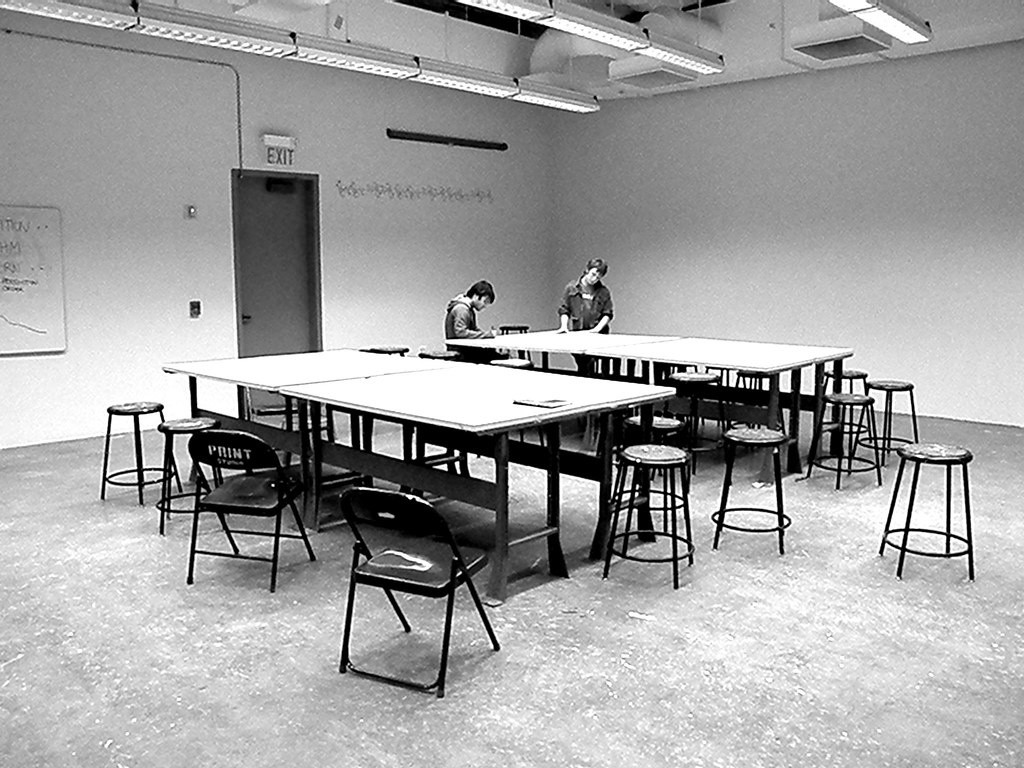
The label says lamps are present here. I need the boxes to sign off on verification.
[405,11,519,99]
[828,0,878,15]
[457,0,554,22]
[635,0,726,75]
[125,0,297,58]
[0,0,138,31]
[283,0,420,81]
[535,0,650,52]
[505,20,601,114]
[852,0,934,45]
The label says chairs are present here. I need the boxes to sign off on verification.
[338,486,500,698]
[187,429,316,594]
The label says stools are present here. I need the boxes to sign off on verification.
[726,370,787,435]
[702,366,751,430]
[490,359,545,448]
[499,325,531,361]
[823,370,874,444]
[418,351,481,459]
[684,364,706,428]
[711,429,792,555]
[100,401,183,505]
[807,394,882,490]
[602,445,695,590]
[879,442,976,581]
[360,347,415,433]
[609,416,681,534]
[852,381,919,467]
[155,418,229,536]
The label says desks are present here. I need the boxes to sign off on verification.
[280,364,677,608]
[584,338,853,484]
[444,330,685,384]
[161,349,473,531]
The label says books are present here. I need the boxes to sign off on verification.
[513,397,573,409]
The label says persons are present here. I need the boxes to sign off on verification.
[557,258,614,373]
[445,279,509,363]
